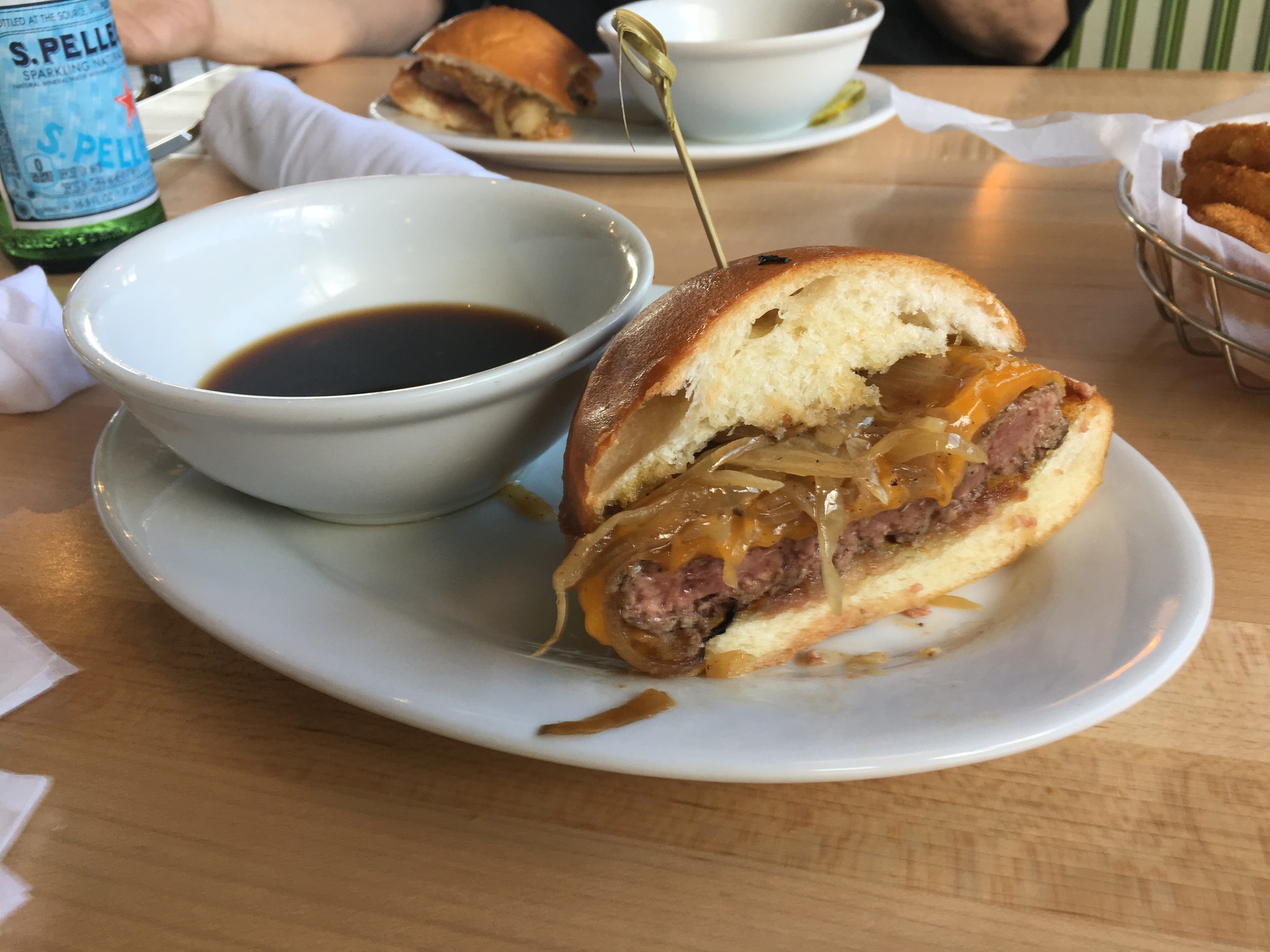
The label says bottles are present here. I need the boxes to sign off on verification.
[0,0,166,272]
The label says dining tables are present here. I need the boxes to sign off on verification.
[0,55,1270,952]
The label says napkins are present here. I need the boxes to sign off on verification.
[0,261,103,415]
[195,65,512,196]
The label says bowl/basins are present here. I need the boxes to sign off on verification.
[596,0,885,138]
[61,172,656,526]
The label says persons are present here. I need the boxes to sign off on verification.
[108,0,1093,68]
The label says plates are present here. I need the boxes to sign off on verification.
[371,54,904,175]
[88,285,1214,784]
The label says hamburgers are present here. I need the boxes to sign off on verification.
[528,242,1112,683]
[385,7,602,137]
